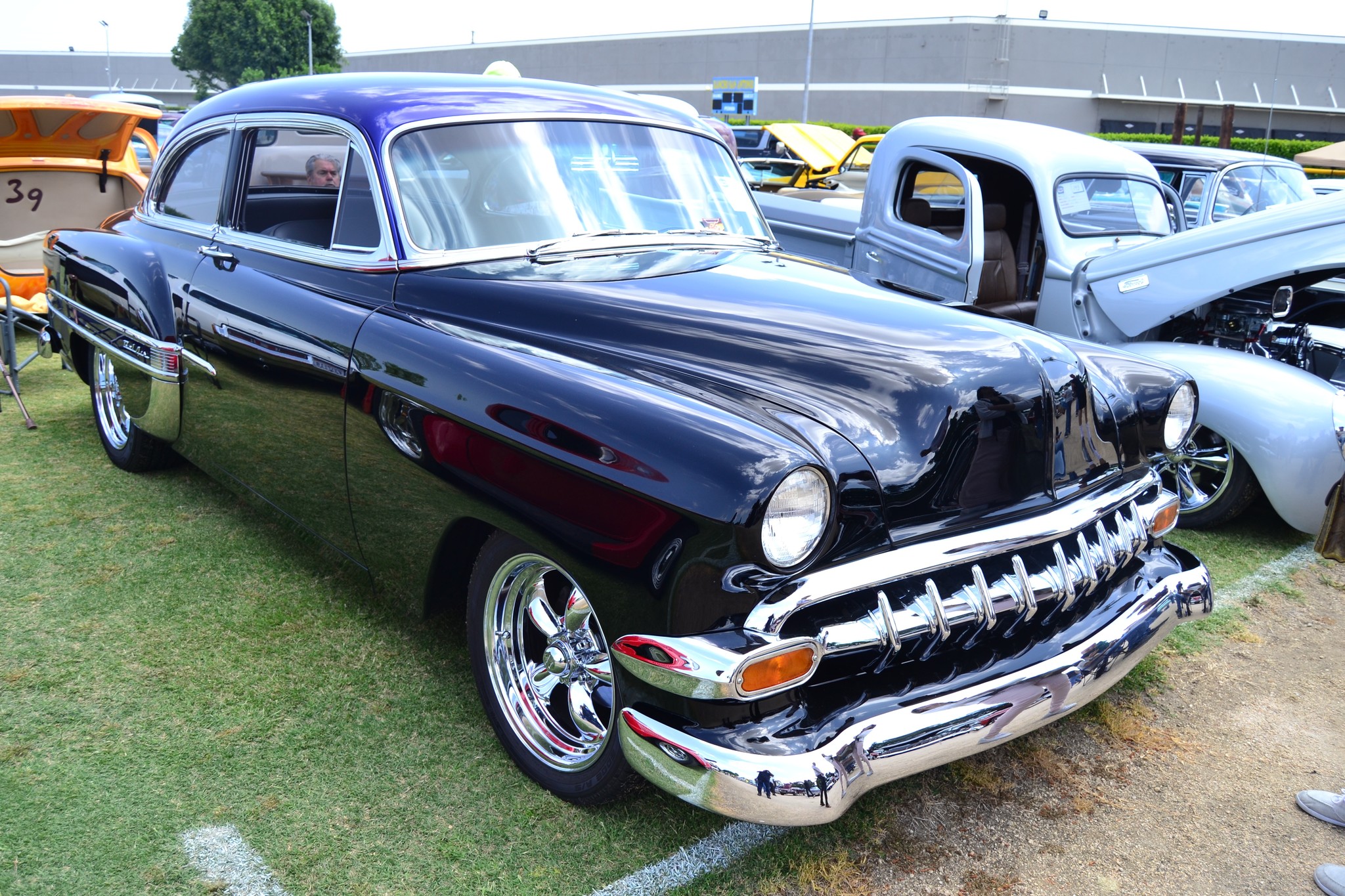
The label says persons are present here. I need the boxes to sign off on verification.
[305,153,342,187]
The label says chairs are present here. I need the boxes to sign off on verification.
[901,193,1038,323]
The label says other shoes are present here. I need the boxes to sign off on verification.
[1314,863,1345,896]
[1295,788,1345,827]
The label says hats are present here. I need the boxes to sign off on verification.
[852,128,866,135]
[483,60,521,78]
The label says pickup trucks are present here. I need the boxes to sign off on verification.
[597,115,1345,536]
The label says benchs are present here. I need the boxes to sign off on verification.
[254,216,334,246]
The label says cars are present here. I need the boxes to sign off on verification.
[0,89,472,316]
[37,73,1214,828]
[732,120,1345,293]
